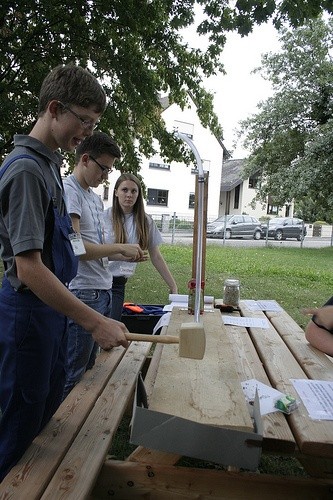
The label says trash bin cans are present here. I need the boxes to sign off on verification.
[313,224,322,237]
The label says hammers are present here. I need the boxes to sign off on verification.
[124,321,206,360]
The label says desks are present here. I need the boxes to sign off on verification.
[99,298,333,500]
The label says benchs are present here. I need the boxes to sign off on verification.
[0,341,154,500]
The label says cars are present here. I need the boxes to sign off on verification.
[206,215,262,240]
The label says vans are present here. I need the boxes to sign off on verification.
[263,217,307,241]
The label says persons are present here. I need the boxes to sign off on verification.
[0,66,130,484]
[62,133,149,403]
[305,295,333,356]
[101,174,178,322]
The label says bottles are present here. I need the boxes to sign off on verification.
[223,279,240,305]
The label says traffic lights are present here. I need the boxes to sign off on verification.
[189,194,195,208]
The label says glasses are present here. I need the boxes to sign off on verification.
[89,156,113,175]
[59,100,97,131]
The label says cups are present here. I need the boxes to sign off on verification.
[187,279,204,315]
